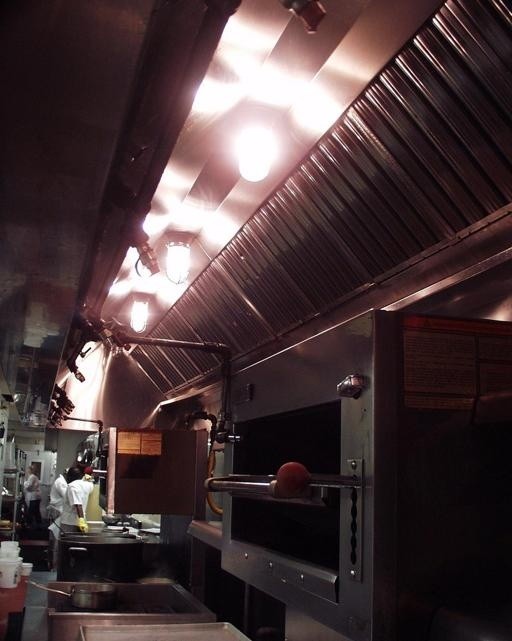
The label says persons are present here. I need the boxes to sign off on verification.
[46,467,71,574]
[21,465,45,532]
[56,467,94,542]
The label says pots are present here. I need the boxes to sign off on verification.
[54,531,146,583]
[22,579,116,610]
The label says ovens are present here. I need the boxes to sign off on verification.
[205,299,512,641]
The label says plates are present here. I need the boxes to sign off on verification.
[87,519,105,533]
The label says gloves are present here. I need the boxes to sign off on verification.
[78,518,88,534]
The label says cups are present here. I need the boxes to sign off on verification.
[0,540,34,589]
